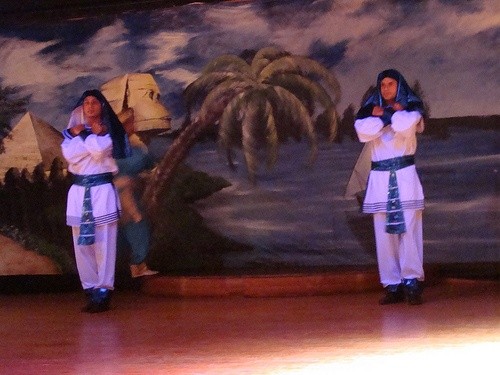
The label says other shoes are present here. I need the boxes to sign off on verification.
[409,288,422,305]
[89,288,111,313]
[82,288,99,312]
[130,263,160,279]
[380,288,404,304]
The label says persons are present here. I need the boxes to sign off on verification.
[354,70,425,306]
[60,90,160,313]
